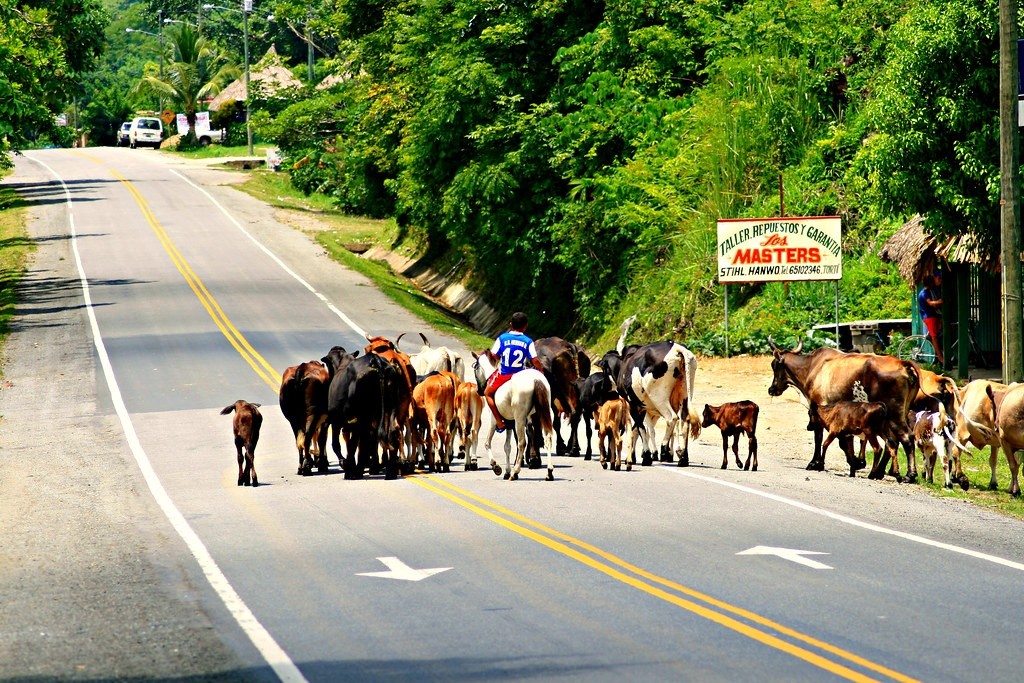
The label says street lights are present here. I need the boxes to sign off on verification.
[202,0,253,157]
[163,0,202,37]
[126,9,163,118]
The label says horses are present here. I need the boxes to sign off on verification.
[469,350,555,480]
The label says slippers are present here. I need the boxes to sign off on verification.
[495,424,504,433]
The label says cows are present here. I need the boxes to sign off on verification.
[702,400,760,471]
[219,400,264,486]
[763,333,1024,497]
[278,331,483,481]
[528,335,696,472]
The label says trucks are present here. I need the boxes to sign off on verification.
[176,111,227,148]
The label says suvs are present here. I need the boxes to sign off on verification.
[117,122,132,147]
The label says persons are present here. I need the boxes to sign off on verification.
[484,312,544,429]
[917,274,943,363]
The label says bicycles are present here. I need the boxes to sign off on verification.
[897,317,990,371]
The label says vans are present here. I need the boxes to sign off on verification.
[128,117,164,149]
